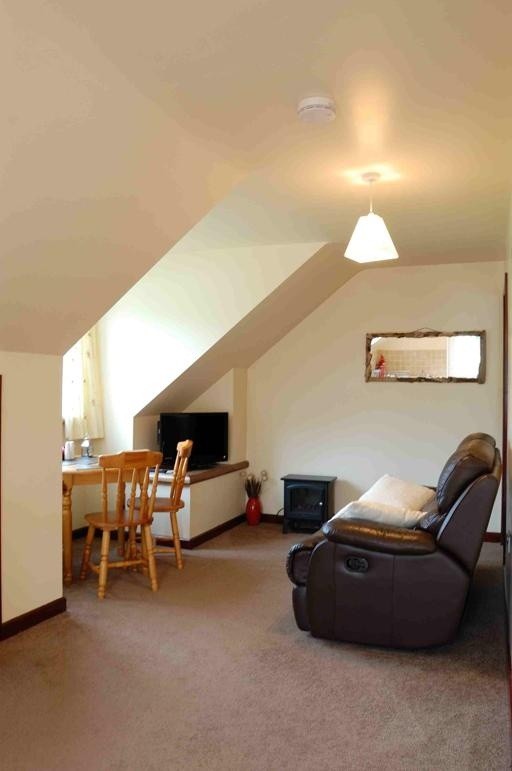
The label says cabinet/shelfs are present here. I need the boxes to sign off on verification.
[280,474,337,534]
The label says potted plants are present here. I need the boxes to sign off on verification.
[244,475,263,527]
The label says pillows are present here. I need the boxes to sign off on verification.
[329,501,423,529]
[359,474,432,511]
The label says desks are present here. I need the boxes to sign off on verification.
[62,455,146,588]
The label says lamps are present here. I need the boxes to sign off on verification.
[342,173,399,264]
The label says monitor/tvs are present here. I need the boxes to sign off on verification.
[158,411,229,471]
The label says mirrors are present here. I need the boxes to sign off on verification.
[367,333,487,384]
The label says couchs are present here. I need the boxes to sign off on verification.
[285,433,502,649]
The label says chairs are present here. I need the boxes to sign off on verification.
[77,448,163,598]
[128,440,194,576]
